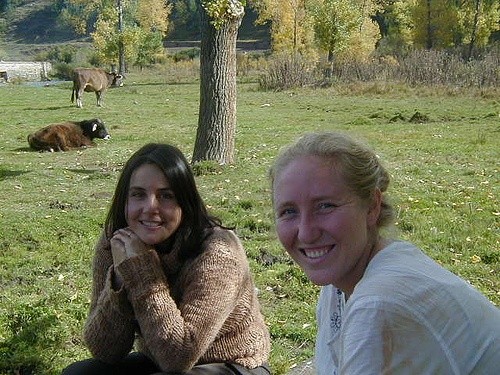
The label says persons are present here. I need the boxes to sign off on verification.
[271,132,500,375]
[63,142,275,375]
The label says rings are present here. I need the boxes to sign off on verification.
[129,232,135,237]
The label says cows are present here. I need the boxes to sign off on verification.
[27,118,112,152]
[71,67,126,108]
